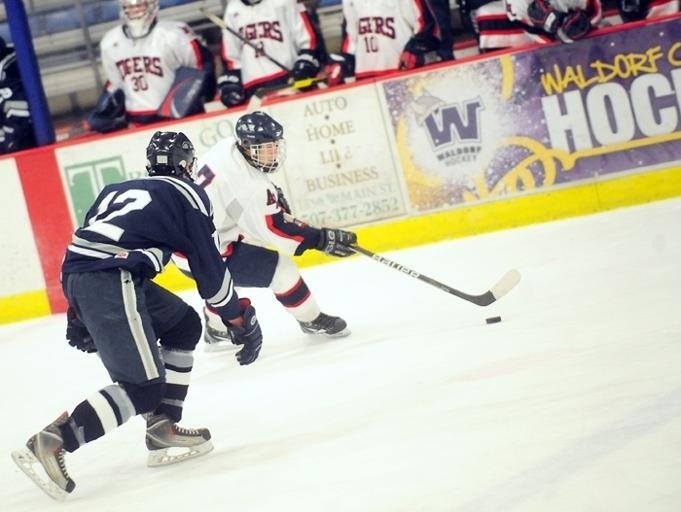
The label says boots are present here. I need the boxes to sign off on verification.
[140,414,217,469]
[202,306,234,347]
[7,409,89,504]
[296,312,354,339]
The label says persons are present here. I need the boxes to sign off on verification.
[170,110,357,349]
[464,0,602,53]
[218,0,325,113]
[316,1,454,90]
[76,0,215,129]
[0,38,35,155]
[10,131,263,501]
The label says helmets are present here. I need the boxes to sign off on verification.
[116,1,163,40]
[235,109,293,176]
[145,126,203,183]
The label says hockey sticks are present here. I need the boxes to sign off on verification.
[282,210,522,307]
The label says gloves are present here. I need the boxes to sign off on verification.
[314,223,361,261]
